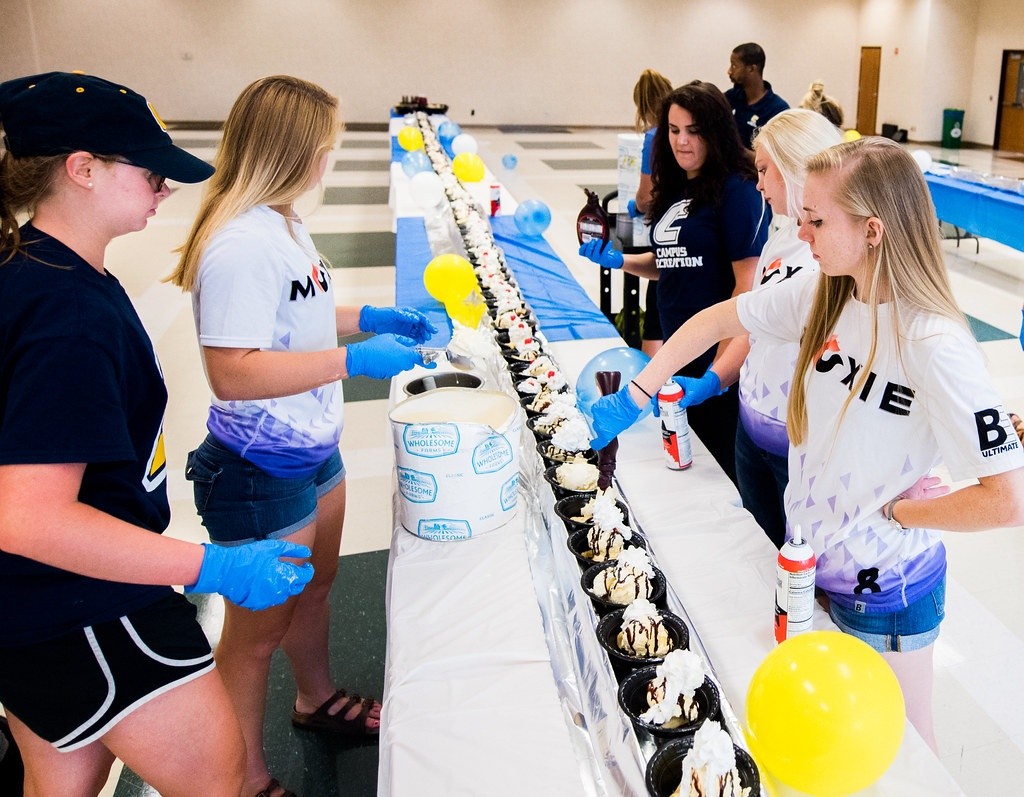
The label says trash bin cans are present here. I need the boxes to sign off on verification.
[941,108,965,148]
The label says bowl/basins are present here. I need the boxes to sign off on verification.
[417,111,762,797]
[403,372,486,395]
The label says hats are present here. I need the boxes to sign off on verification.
[0,71,216,184]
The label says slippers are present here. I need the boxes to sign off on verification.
[255,778,297,797]
[291,690,380,735]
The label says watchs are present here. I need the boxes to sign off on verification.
[888,497,910,536]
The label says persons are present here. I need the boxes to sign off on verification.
[596,133,1023,753]
[578,81,769,480]
[724,40,791,172]
[626,69,674,218]
[0,72,313,797]
[801,81,844,125]
[161,75,439,797]
[647,108,844,550]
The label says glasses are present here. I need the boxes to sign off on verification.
[90,153,164,194]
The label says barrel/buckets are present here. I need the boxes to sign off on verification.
[389,387,521,541]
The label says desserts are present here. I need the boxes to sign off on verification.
[415,112,760,797]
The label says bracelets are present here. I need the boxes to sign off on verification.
[631,380,652,400]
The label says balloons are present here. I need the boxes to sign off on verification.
[514,199,551,236]
[911,149,932,174]
[438,121,462,144]
[451,134,485,181]
[402,150,446,207]
[398,126,422,152]
[424,254,484,326]
[577,346,658,423]
[843,130,860,142]
[503,154,517,168]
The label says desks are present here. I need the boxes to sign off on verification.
[923,170,1024,254]
[377,106,962,797]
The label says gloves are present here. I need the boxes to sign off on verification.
[651,371,721,418]
[346,333,437,380]
[186,540,318,611]
[579,239,624,270]
[359,305,438,344]
[626,200,645,219]
[589,384,642,451]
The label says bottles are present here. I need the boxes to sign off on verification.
[775,522,816,643]
[658,377,693,470]
[490,185,501,216]
[577,188,610,250]
